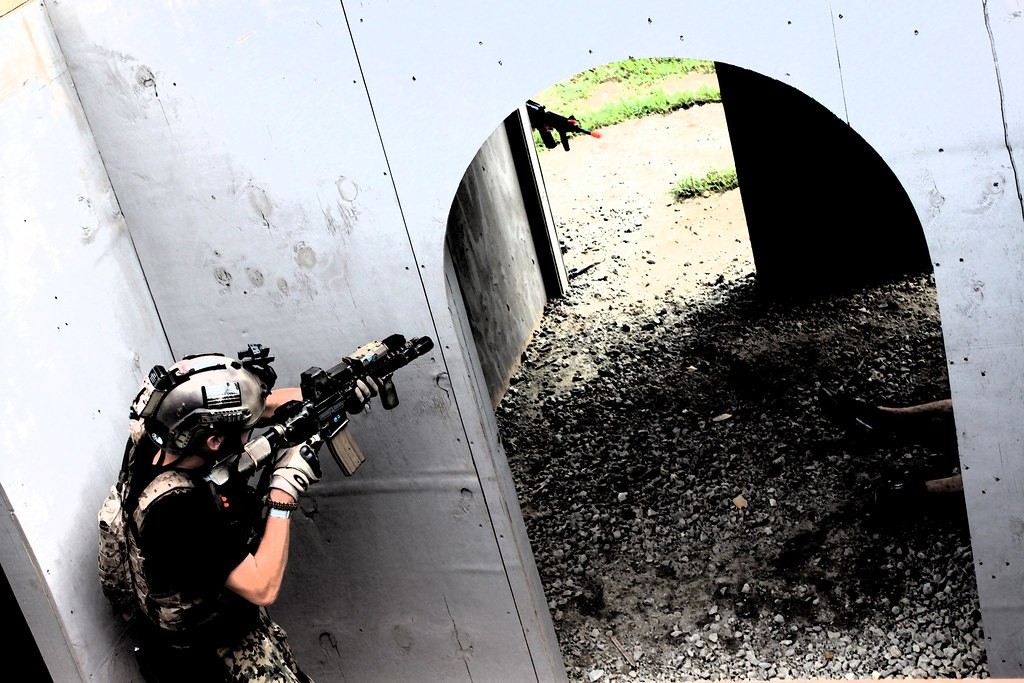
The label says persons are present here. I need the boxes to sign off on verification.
[116,349,386,683]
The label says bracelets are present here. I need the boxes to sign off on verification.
[262,491,298,512]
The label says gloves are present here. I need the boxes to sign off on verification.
[268,432,321,500]
[344,375,379,415]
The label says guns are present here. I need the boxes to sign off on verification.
[205,332,437,501]
[526,100,603,154]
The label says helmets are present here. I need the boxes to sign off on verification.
[144,353,267,455]
